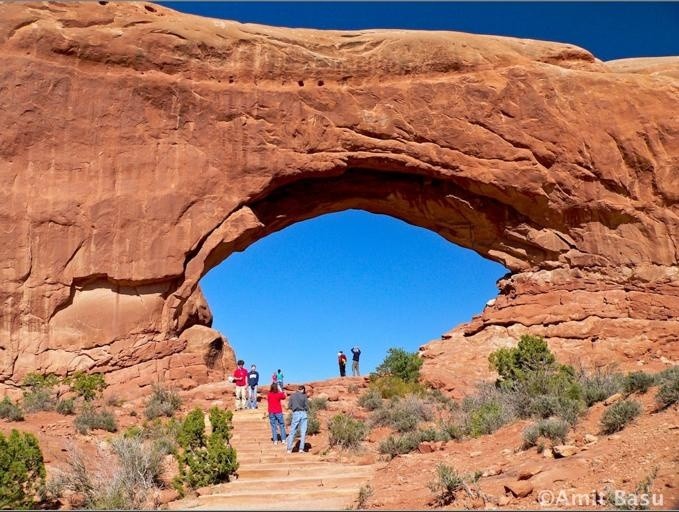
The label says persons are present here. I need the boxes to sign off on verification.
[245,364,259,410]
[232,360,250,411]
[286,385,310,455]
[273,369,284,390]
[267,383,287,445]
[337,351,347,377]
[351,346,360,376]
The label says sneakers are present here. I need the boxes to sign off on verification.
[287,450,292,454]
[273,440,286,445]
[299,449,304,452]
